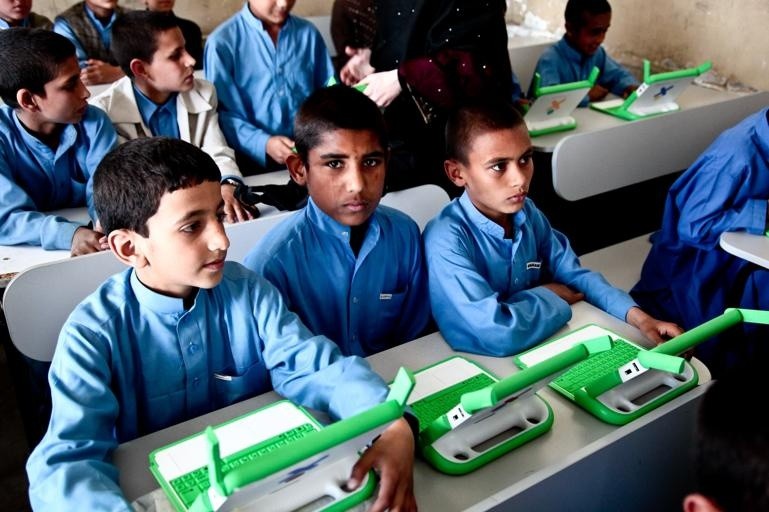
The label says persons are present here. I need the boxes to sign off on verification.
[244,84,431,356]
[422,95,694,360]
[680,430,769,512]
[0,0,641,256]
[24,136,419,512]
[628,108,769,462]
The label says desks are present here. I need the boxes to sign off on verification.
[1,0,769,512]
